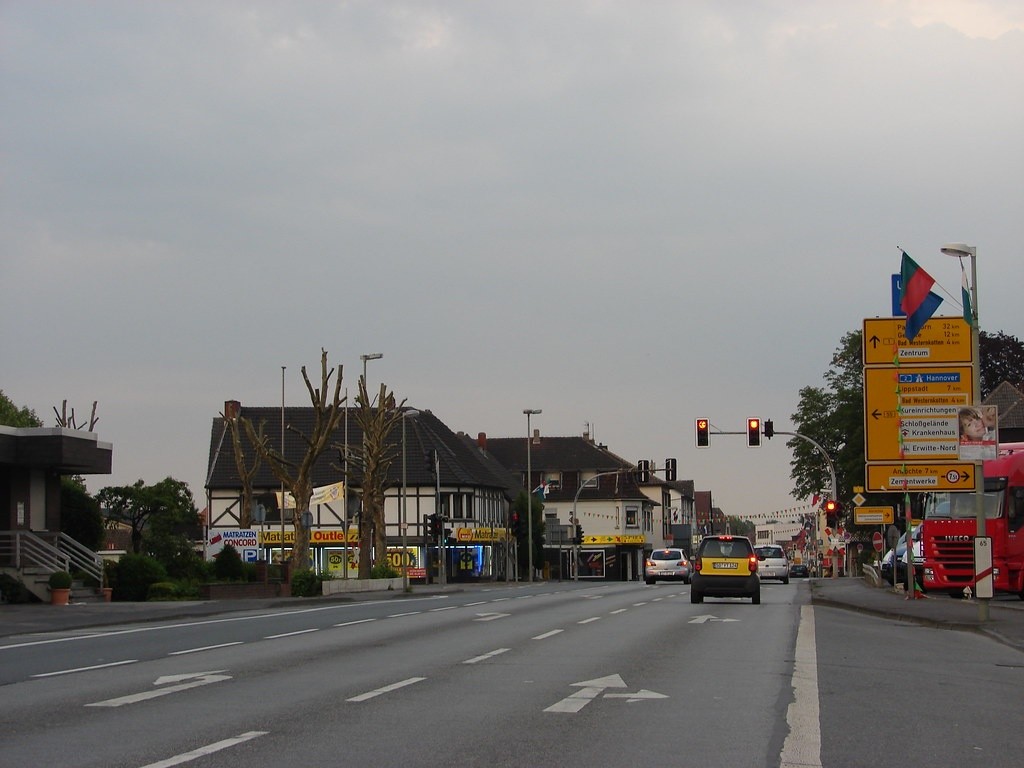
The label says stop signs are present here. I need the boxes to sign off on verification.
[873,532,883,552]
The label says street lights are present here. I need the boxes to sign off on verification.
[402,409,420,592]
[361,354,383,388]
[940,243,991,597]
[523,409,542,581]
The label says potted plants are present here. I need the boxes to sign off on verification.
[49,570,73,606]
[96,565,114,603]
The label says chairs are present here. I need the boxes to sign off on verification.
[732,543,746,554]
[706,542,720,553]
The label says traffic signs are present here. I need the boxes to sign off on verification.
[854,506,895,524]
[861,317,976,492]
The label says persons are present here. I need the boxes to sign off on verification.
[811,565,816,577]
[959,407,997,442]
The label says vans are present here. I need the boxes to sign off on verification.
[901,501,951,591]
[879,524,915,587]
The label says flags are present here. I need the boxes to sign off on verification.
[899,251,943,342]
[961,265,973,325]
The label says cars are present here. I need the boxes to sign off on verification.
[690,534,760,605]
[789,565,808,578]
[753,544,789,584]
[643,547,691,585]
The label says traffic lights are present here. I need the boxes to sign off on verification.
[747,418,762,448]
[512,511,521,537]
[826,501,836,528]
[695,418,711,449]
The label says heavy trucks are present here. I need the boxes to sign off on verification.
[921,441,1024,601]
